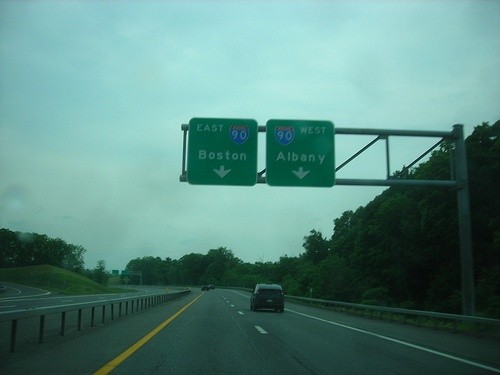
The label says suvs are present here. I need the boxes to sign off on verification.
[250,280,286,313]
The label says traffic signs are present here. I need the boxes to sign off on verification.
[265,119,337,188]
[187,115,259,188]
